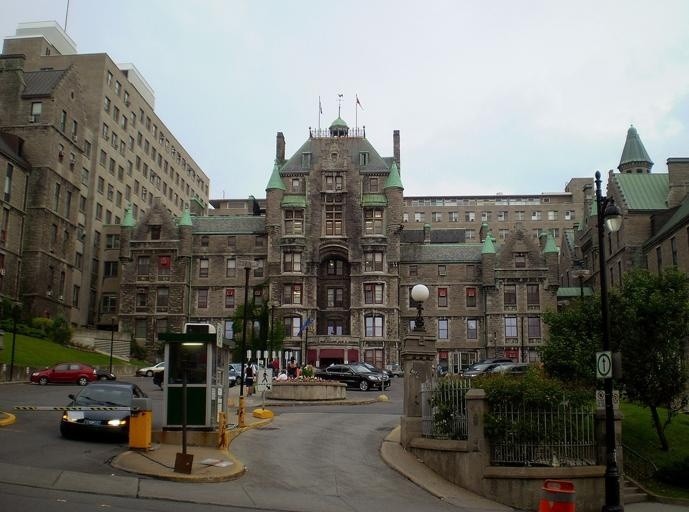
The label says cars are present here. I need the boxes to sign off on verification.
[60,379,149,444]
[459,356,529,378]
[315,361,391,391]
[228,362,261,388]
[136,361,165,377]
[386,364,403,379]
[96,368,116,381]
[30,361,98,385]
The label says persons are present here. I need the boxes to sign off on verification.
[287,356,299,378]
[262,370,268,383]
[273,356,279,373]
[245,361,254,397]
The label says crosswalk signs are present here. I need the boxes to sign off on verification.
[256,368,273,393]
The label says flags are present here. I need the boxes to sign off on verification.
[357,97,364,110]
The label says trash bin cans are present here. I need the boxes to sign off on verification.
[541,479,577,512]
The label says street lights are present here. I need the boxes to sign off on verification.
[10,301,19,380]
[110,316,117,374]
[409,285,429,332]
[597,195,624,512]
[238,262,251,427]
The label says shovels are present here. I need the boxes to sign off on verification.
[175,373,191,474]
